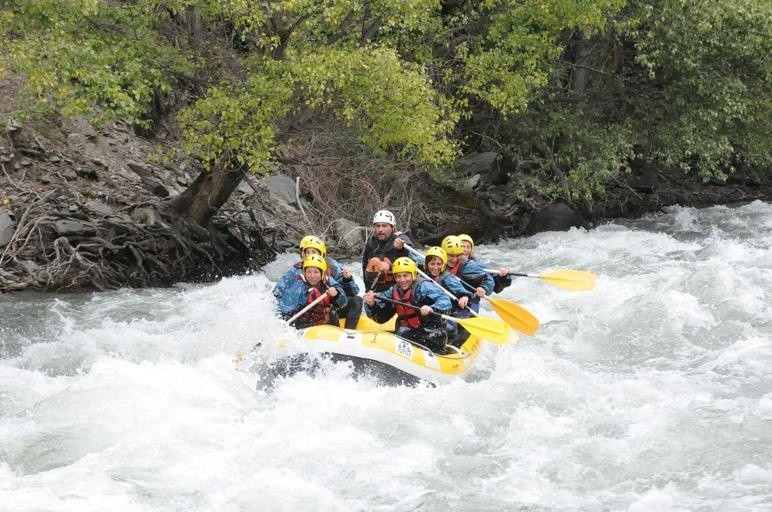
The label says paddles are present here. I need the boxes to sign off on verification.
[374,295,511,346]
[486,269,596,292]
[404,246,540,345]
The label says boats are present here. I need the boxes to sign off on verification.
[260,301,487,394]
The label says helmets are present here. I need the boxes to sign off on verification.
[441,234,465,255]
[303,237,327,258]
[391,256,417,283]
[299,235,314,249]
[458,233,475,258]
[303,254,328,281]
[425,245,448,274]
[373,209,396,226]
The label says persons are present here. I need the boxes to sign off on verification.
[362,209,416,291]
[421,246,474,337]
[273,234,363,332]
[440,234,496,316]
[363,257,452,355]
[458,232,512,293]
